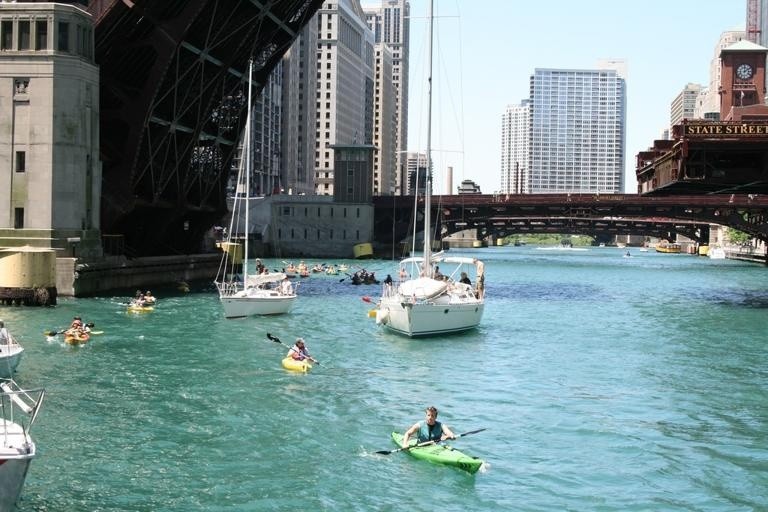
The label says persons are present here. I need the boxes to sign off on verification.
[460,272,471,285]
[442,275,455,293]
[402,405,457,450]
[64,316,95,335]
[286,338,311,361]
[0,318,13,344]
[432,266,443,282]
[133,289,155,307]
[254,257,393,295]
[472,257,485,301]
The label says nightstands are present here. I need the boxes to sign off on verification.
[374,428,486,455]
[44,329,103,335]
[266,332,328,370]
[339,269,364,282]
[361,296,377,305]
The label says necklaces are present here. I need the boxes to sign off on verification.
[212,48,302,319]
[370,0,491,341]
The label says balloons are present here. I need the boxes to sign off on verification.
[735,63,753,81]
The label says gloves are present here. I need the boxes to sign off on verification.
[281,355,312,374]
[282,265,377,286]
[390,430,483,475]
[639,248,649,252]
[128,304,155,312]
[64,332,90,346]
[0,317,26,379]
[654,242,682,254]
[0,379,48,512]
